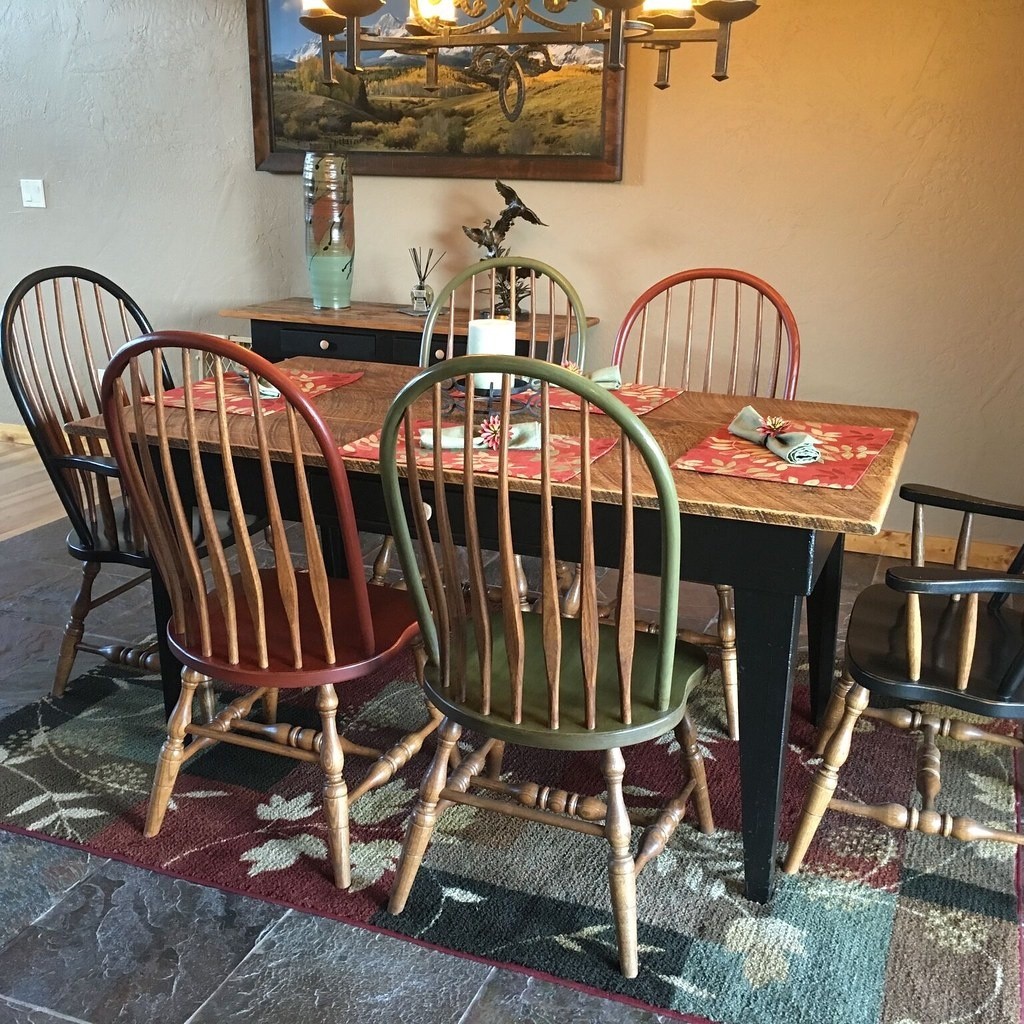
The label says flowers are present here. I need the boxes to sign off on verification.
[561,360,581,374]
[756,415,792,439]
[477,415,514,452]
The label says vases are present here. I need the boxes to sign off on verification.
[302,152,356,311]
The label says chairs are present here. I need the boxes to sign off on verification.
[369,256,566,611]
[563,269,801,741]
[784,483,1024,874]
[378,356,716,979]
[100,329,465,889]
[0,264,274,696]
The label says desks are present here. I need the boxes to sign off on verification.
[60,354,920,907]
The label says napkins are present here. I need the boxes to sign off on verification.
[549,365,622,391]
[419,421,541,451]
[727,405,823,465]
[233,361,282,400]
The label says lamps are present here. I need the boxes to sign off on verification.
[299,0,760,122]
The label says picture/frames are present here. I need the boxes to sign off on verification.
[246,0,629,182]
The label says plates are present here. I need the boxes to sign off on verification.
[397,306,446,317]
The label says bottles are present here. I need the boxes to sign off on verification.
[409,281,433,312]
[302,150,355,312]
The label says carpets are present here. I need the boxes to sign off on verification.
[0,647,1024,1024]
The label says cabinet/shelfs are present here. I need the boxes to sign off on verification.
[220,297,599,374]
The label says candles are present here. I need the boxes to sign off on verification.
[467,319,516,390]
